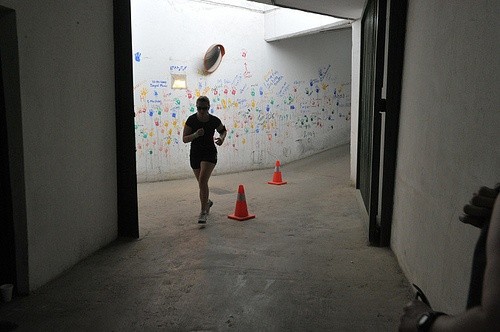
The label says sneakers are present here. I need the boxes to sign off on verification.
[197,212,209,223]
[206,199,213,213]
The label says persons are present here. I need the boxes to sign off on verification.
[183,96,227,223]
[399,192,500,332]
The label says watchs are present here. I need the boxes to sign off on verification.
[416,311,448,332]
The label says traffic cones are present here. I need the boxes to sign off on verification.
[228,185,255,221]
[268,161,287,185]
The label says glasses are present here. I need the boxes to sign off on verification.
[196,106,210,110]
